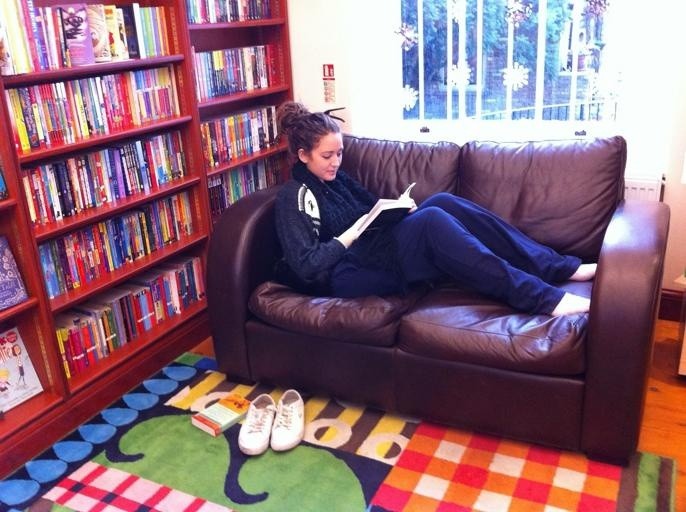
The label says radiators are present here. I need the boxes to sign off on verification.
[623,172,666,200]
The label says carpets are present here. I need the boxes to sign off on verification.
[0,351,678,512]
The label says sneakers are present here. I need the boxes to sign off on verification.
[270,389,305,453]
[238,393,276,456]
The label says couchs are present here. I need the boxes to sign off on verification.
[206,133,671,467]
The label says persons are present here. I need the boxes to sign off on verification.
[273,102,599,318]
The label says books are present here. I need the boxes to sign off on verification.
[0,0,182,153]
[187,0,284,223]
[21,130,194,299]
[53,254,205,377]
[353,181,418,244]
[0,169,43,413]
[191,394,251,437]
